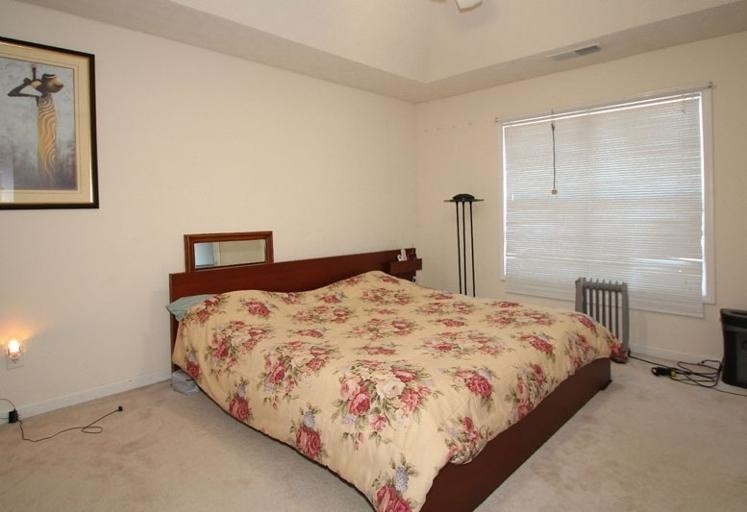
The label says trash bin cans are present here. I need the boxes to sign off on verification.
[719,308,747,389]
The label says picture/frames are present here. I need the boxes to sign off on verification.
[0,36,101,211]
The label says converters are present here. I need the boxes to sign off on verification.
[9,410,18,423]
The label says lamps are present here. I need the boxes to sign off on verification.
[6,339,22,362]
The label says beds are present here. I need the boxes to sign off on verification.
[167,246,624,512]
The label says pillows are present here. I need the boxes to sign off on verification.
[165,293,219,321]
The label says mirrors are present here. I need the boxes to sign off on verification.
[182,230,275,273]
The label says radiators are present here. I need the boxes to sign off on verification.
[572,273,630,363]
